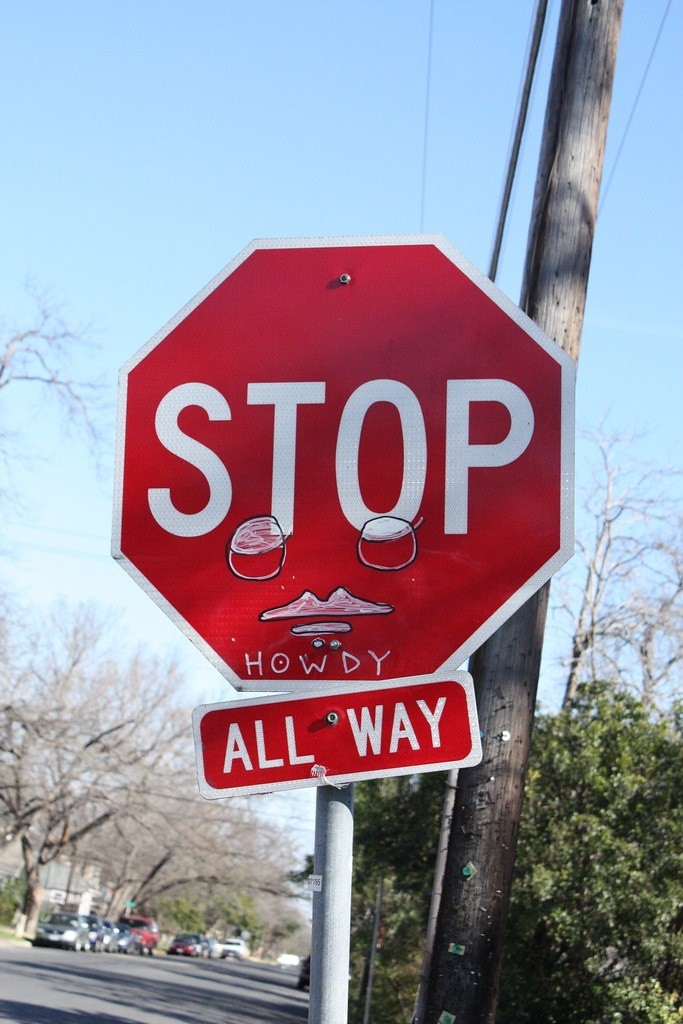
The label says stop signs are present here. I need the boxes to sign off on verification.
[103,232,576,702]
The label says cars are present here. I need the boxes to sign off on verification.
[32,902,252,961]
